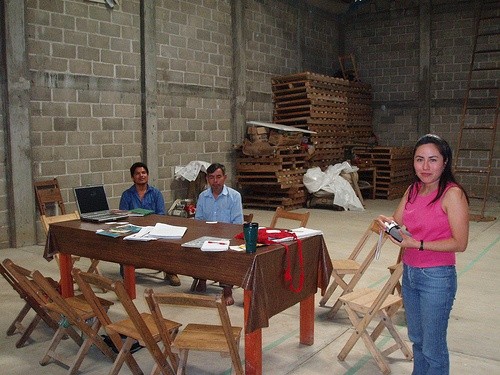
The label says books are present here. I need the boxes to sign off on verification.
[124,207,156,216]
[201,240,230,251]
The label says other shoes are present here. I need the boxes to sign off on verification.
[164,272,181,286]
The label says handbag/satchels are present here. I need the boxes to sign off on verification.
[235,227,304,293]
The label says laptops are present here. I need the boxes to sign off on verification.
[73,183,129,223]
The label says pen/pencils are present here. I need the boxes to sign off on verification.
[262,244,270,246]
[206,221,217,224]
[208,242,227,245]
[140,232,150,238]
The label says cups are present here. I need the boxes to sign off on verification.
[243,223,258,253]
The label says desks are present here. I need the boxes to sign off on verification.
[43,208,334,375]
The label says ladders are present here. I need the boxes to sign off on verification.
[452,0,500,218]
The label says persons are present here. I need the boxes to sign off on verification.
[194,163,244,305]
[119,162,180,286]
[376,133,470,375]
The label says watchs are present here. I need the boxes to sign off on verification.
[417,239,424,251]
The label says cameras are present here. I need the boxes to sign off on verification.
[384,221,397,232]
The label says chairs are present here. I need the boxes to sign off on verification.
[0,177,413,375]
